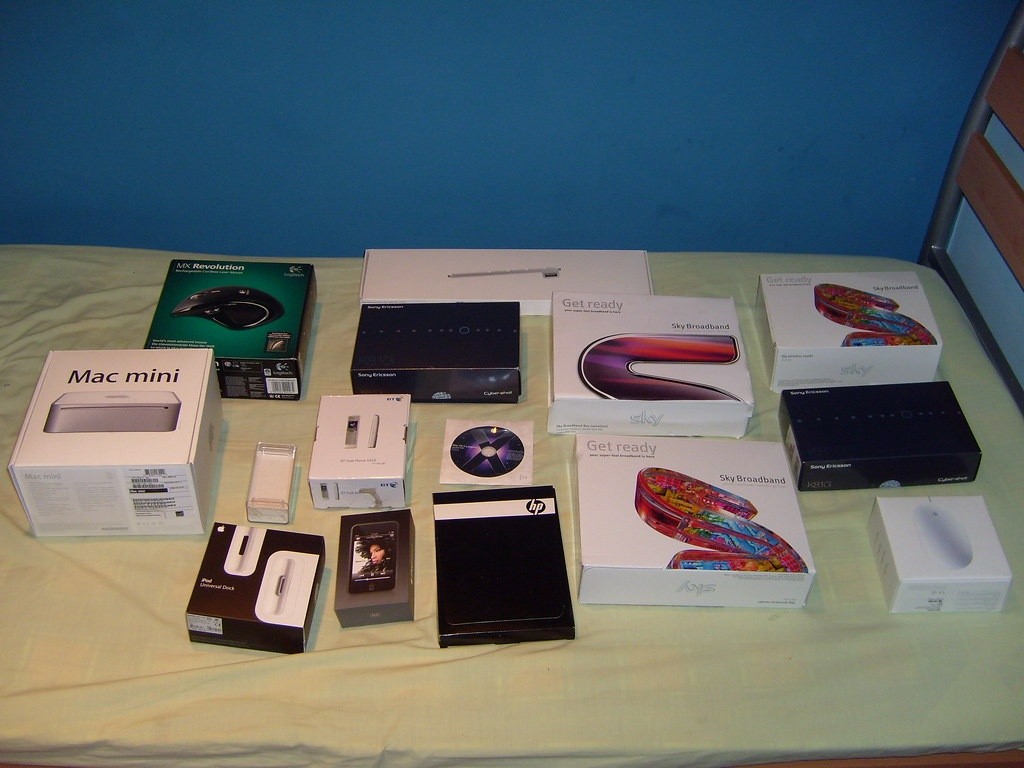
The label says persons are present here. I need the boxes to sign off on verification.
[353,534,395,578]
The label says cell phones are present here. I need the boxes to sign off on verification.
[348,521,398,593]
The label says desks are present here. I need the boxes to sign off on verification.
[0,245,1024,768]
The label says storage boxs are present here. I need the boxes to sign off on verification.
[185,522,326,655]
[359,248,654,316]
[334,509,416,629]
[865,495,1013,614]
[778,381,983,491]
[432,485,576,648]
[308,393,411,510]
[753,272,943,393]
[546,291,755,440]
[246,441,298,525]
[8,349,225,537]
[142,259,317,402]
[570,432,817,610]
[350,302,522,404]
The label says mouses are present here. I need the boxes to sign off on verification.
[914,504,974,570]
[171,285,286,330]
